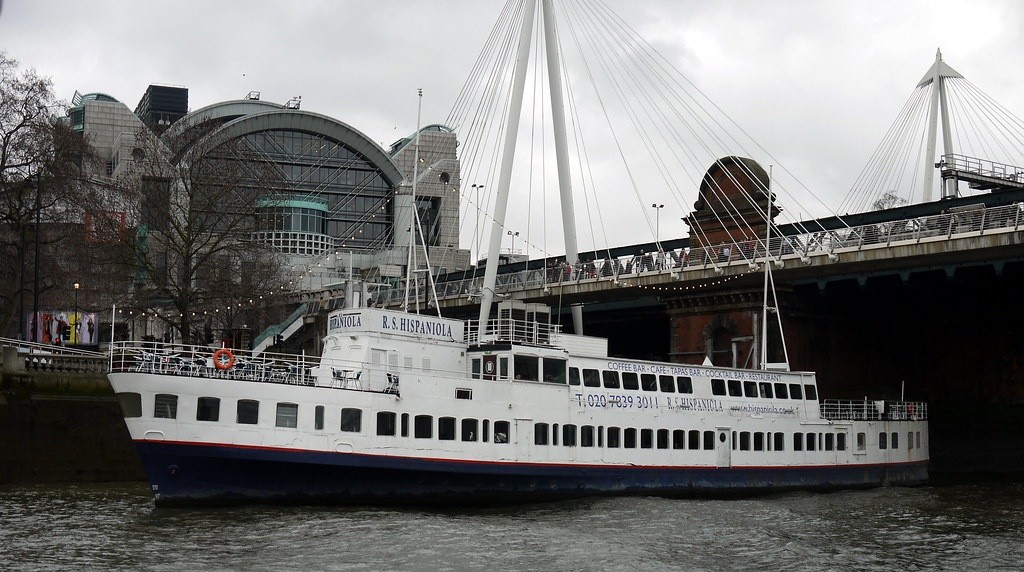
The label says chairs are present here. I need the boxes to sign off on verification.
[129,350,399,395]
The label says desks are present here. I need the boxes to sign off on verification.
[341,368,354,389]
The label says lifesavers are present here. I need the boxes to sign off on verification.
[214,350,234,369]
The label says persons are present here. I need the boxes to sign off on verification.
[55,316,67,338]
[76,318,82,341]
[44,316,53,341]
[701,246,710,265]
[738,236,753,260]
[600,257,610,277]
[717,241,727,262]
[679,248,688,267]
[633,250,654,272]
[87,318,94,342]
[547,254,596,282]
[657,248,663,271]
[625,261,632,274]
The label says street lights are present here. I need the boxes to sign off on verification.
[507,231,519,263]
[74,282,80,347]
[472,184,485,270]
[651,203,664,244]
[33,166,42,343]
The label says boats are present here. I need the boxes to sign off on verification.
[104,0,931,507]
